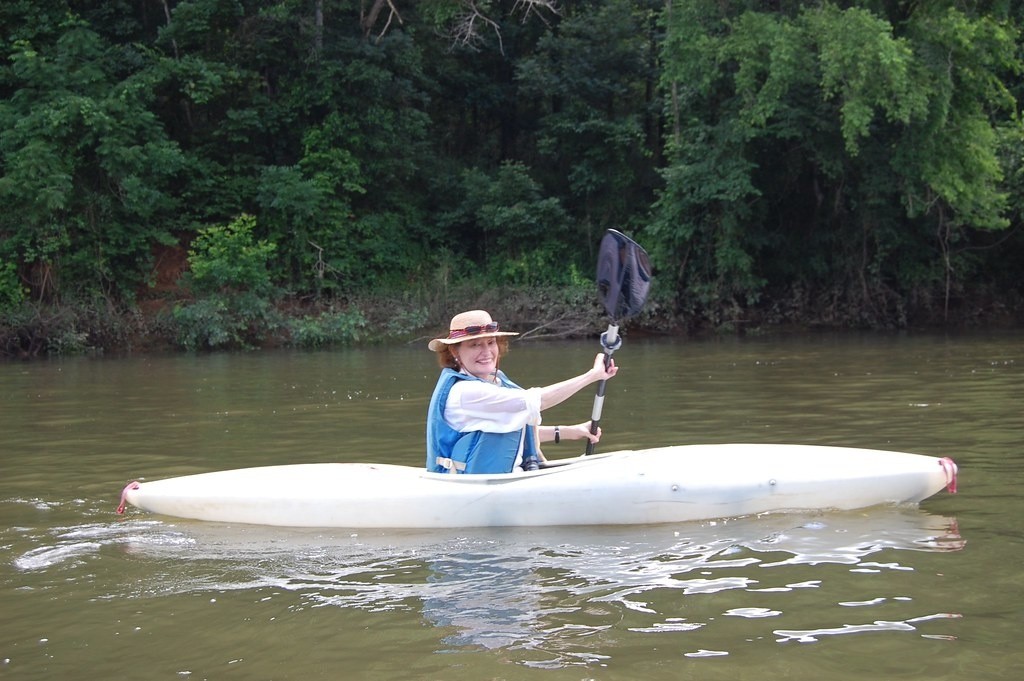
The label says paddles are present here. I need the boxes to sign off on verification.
[586,224,652,458]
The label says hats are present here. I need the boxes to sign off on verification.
[428,310,520,352]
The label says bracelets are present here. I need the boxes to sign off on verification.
[555,425,559,443]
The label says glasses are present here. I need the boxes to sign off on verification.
[449,322,499,335]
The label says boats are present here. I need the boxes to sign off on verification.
[134,503,968,566]
[117,441,959,529]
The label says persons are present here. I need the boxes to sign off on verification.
[426,310,619,474]
